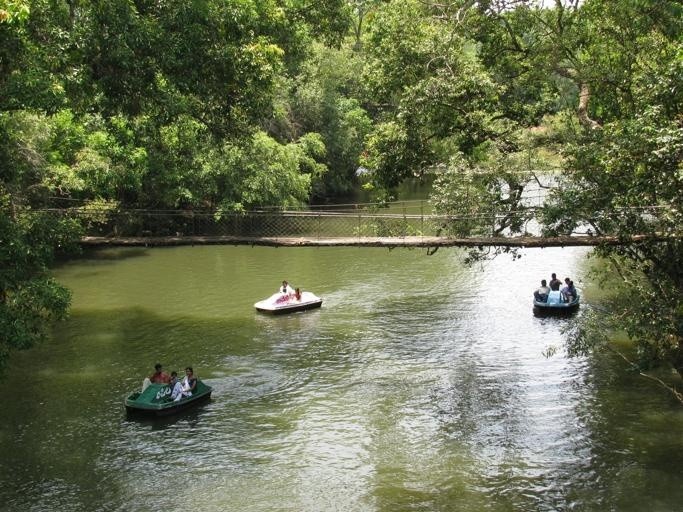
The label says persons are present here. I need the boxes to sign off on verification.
[561,281,576,301]
[286,288,302,305]
[280,281,292,293]
[565,278,576,288]
[171,367,197,402]
[534,279,550,302]
[169,372,177,386]
[549,273,562,291]
[150,364,170,384]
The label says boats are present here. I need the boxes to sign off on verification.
[254,292,324,315]
[124,379,215,418]
[533,291,580,312]
